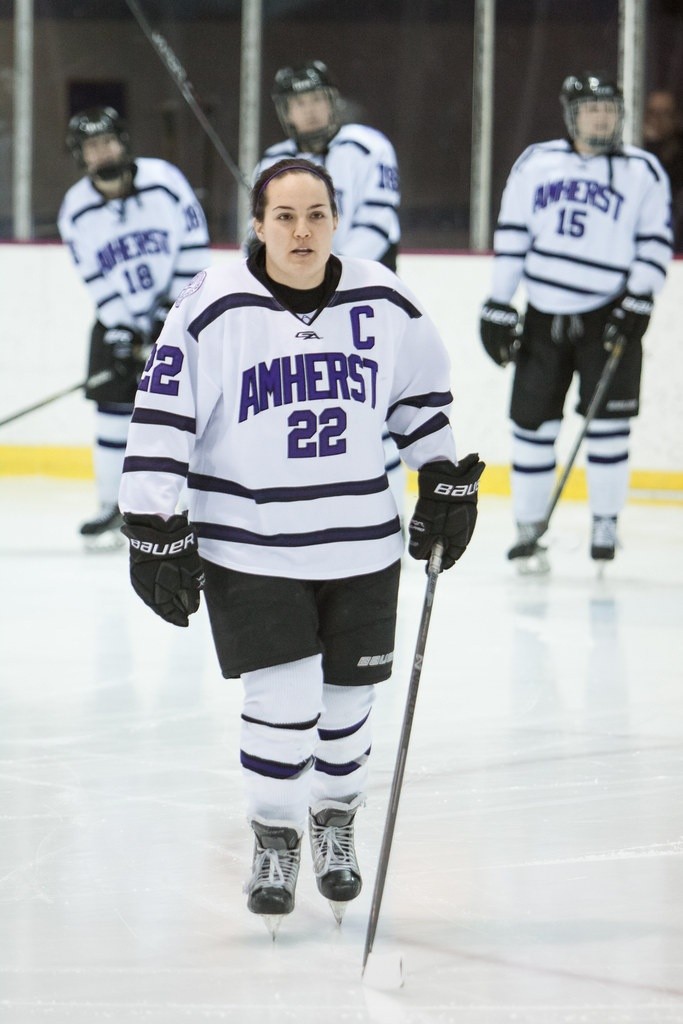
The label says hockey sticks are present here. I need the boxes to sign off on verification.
[357,536,446,979]
[504,332,625,563]
[0,366,118,427]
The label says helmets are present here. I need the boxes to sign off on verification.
[270,59,340,147]
[64,105,134,181]
[558,71,625,153]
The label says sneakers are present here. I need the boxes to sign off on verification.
[508,523,551,574]
[590,514,617,559]
[308,793,367,927]
[240,812,304,944]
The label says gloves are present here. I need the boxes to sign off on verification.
[112,325,144,381]
[603,290,654,356]
[151,297,174,344]
[479,298,521,368]
[408,453,486,576]
[121,509,208,628]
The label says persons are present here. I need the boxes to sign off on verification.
[118,157,485,940]
[480,73,671,578]
[57,105,209,555]
[245,61,399,276]
